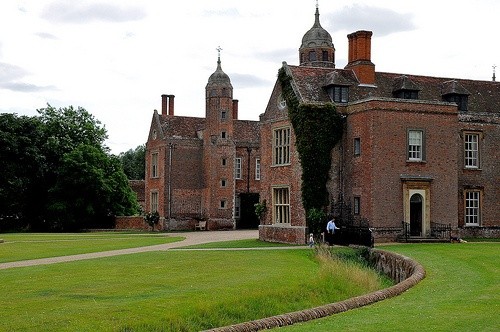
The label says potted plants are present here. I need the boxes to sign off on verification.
[254,201,271,241]
[195,216,210,228]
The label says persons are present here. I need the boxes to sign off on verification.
[327,217,340,245]
[309,233,314,248]
